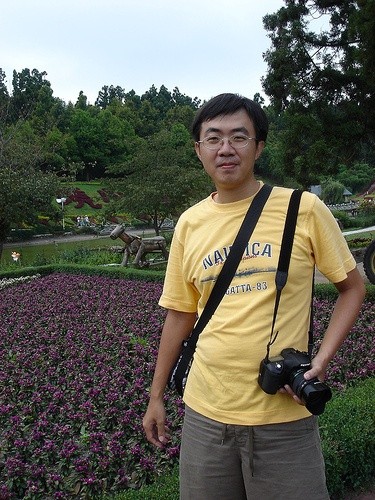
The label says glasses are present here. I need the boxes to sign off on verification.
[198,134,258,150]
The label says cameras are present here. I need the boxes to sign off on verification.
[258,347,332,415]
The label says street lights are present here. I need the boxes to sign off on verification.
[61,197,67,230]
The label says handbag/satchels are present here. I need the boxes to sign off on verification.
[168,337,196,397]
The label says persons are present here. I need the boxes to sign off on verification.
[77,215,89,227]
[143,93,367,500]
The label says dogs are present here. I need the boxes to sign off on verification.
[110,223,169,270]
